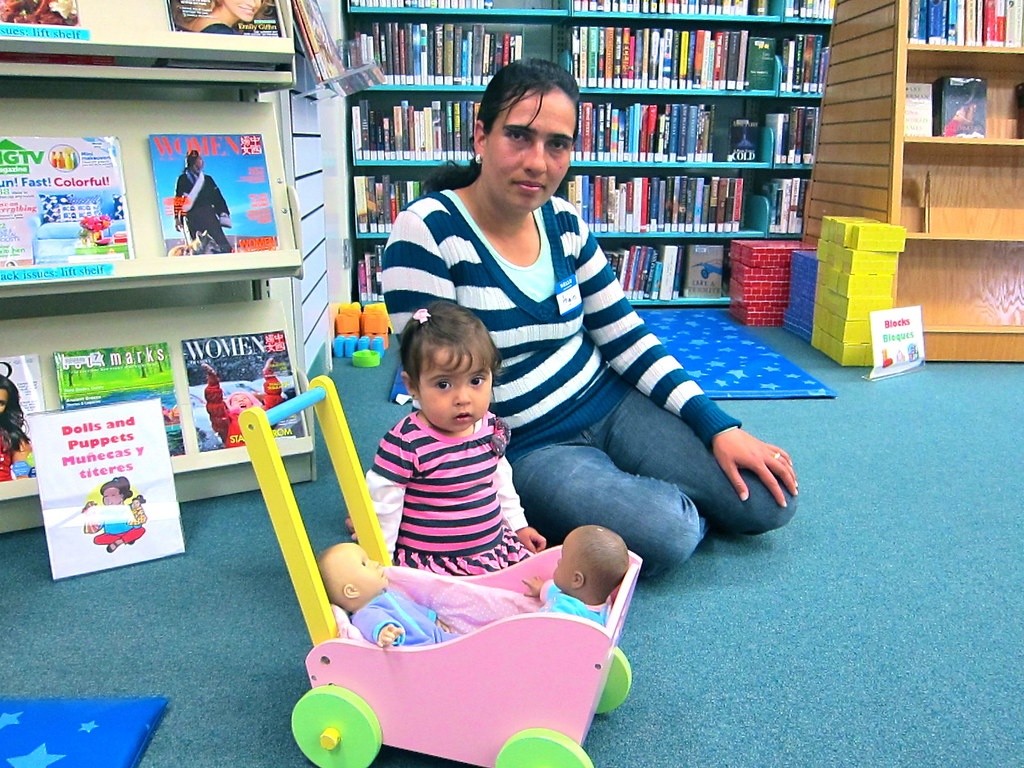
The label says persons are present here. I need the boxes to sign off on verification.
[522,525,629,627]
[365,302,547,576]
[316,543,463,647]
[0,137,134,267]
[346,57,800,576]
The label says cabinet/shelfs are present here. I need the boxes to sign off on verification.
[342,0,833,307]
[802,0,1024,363]
[0,0,320,532]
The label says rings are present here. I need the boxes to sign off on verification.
[773,452,781,459]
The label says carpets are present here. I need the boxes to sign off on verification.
[0,695,169,768]
[392,308,837,401]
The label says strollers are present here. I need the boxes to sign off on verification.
[239,375,646,768]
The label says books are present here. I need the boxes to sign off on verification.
[358,244,386,306]
[0,354,47,483]
[569,102,821,165]
[566,175,809,234]
[354,174,427,233]
[291,0,386,98]
[181,330,310,453]
[908,0,1024,48]
[348,0,835,94]
[352,99,481,162]
[148,135,280,257]
[0,0,78,26]
[904,76,988,139]
[603,244,723,300]
[53,343,186,457]
[167,0,286,39]
[26,397,187,580]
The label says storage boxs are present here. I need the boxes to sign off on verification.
[936,75,987,136]
[728,218,905,368]
[901,82,933,138]
